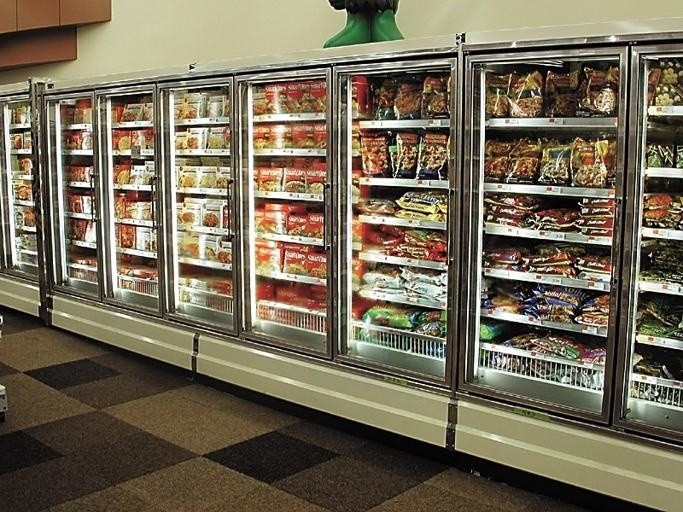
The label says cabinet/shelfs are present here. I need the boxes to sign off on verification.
[456,49,622,425]
[234,67,333,361]
[0,93,47,285]
[612,39,682,444]
[93,83,168,319]
[42,91,105,301]
[155,75,241,337]
[334,58,463,397]
